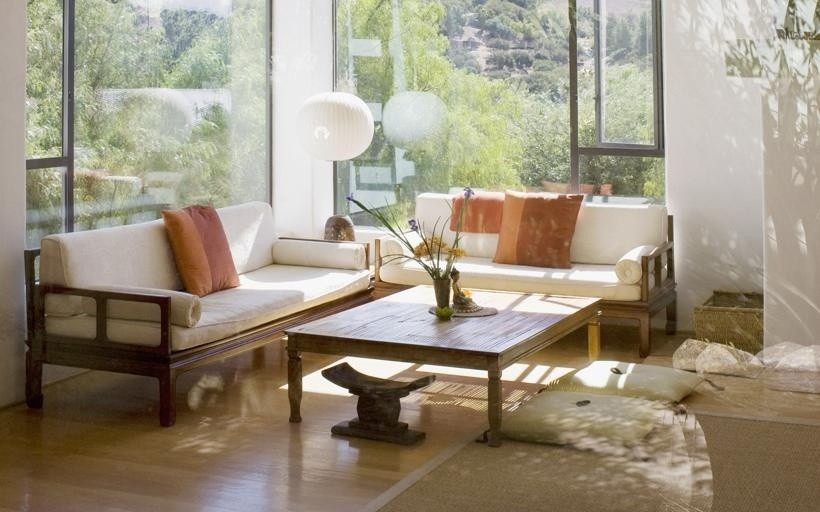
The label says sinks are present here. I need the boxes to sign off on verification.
[24,202,376,426]
[373,192,678,358]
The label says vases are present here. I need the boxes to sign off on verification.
[346,183,475,278]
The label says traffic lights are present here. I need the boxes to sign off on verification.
[271,240,367,270]
[380,231,430,263]
[614,244,654,285]
[161,203,242,296]
[543,181,594,201]
[81,285,203,329]
[537,360,725,415]
[491,189,585,268]
[475,384,668,460]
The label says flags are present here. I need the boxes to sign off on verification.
[435,278,451,308]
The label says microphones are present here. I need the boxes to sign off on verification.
[321,362,436,446]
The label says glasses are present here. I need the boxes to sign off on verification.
[382,91,448,198]
[293,92,374,240]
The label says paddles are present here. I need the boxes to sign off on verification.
[375,413,820,512]
